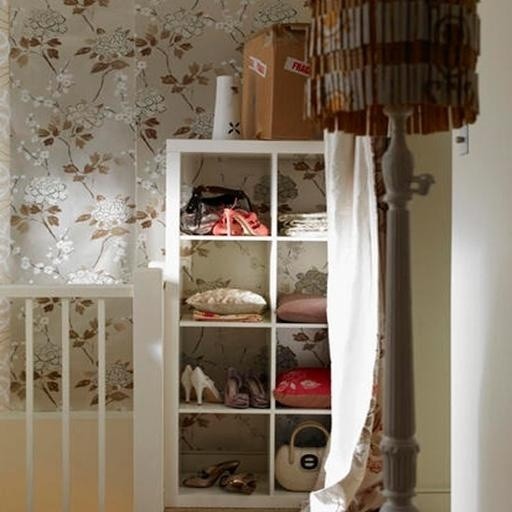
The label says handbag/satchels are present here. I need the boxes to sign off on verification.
[276,445,325,492]
[181,185,253,235]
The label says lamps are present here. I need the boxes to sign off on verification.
[309,2,479,512]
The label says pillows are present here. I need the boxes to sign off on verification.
[184,286,268,316]
[278,292,328,325]
[273,366,332,408]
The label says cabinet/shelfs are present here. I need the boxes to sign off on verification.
[165,138,333,506]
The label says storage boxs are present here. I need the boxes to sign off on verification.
[240,20,325,137]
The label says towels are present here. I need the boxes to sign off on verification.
[278,210,328,239]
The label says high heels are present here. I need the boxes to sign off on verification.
[181,364,269,408]
[183,459,240,488]
[212,207,269,235]
